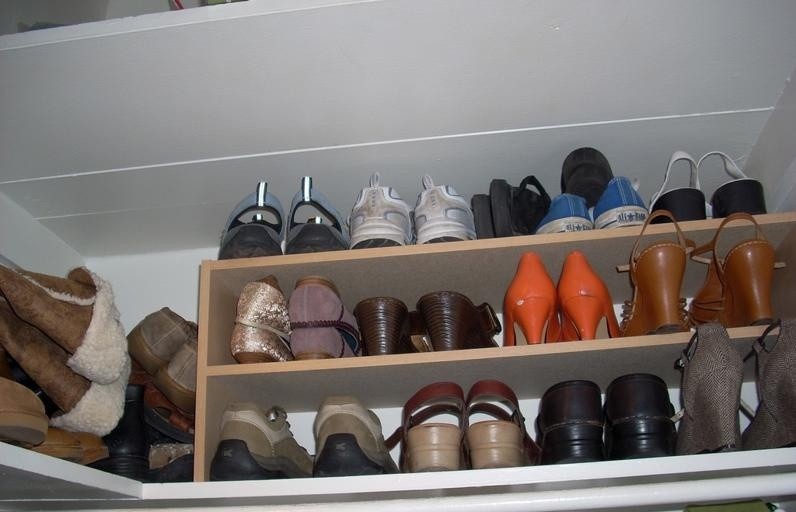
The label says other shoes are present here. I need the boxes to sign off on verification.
[593,177,648,230]
[534,193,591,235]
[562,147,612,207]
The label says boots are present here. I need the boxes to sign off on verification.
[1,265,198,482]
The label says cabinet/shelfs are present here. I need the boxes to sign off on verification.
[194,212,796,483]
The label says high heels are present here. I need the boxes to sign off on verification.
[557,250,622,343]
[533,380,605,466]
[686,212,775,326]
[384,382,466,473]
[603,373,676,460]
[502,253,560,346]
[463,380,543,469]
[617,212,695,335]
[674,323,744,455]
[738,317,796,451]
[416,291,501,351]
[353,297,427,355]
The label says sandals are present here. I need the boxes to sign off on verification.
[284,177,350,256]
[217,181,286,260]
[490,175,552,237]
[471,194,493,239]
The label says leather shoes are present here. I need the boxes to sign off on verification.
[230,274,363,364]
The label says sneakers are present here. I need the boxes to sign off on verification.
[413,173,476,243]
[210,394,400,482]
[347,171,412,249]
